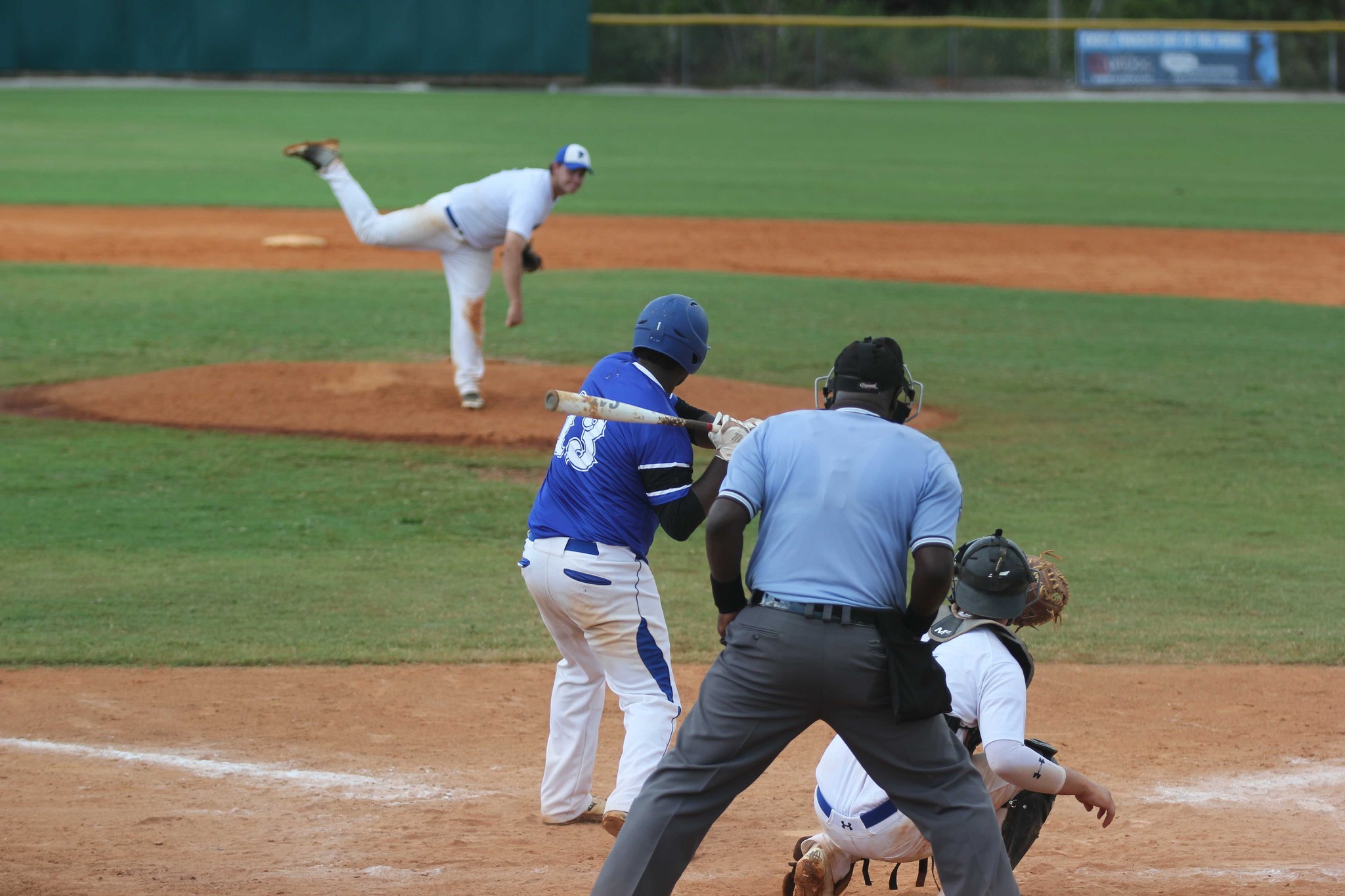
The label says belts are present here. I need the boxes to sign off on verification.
[529,529,598,555]
[753,590,877,625]
[445,207,461,234]
[816,784,901,829]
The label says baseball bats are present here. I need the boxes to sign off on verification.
[543,390,725,436]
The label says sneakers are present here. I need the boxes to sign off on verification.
[460,392,484,409]
[285,140,342,169]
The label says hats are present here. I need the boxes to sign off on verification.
[831,335,905,395]
[556,142,596,175]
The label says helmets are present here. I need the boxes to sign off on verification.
[632,294,711,373]
[955,536,1030,619]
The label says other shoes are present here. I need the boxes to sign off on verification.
[542,795,610,825]
[602,811,631,834]
[793,843,834,896]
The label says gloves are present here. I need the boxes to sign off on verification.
[708,409,768,461]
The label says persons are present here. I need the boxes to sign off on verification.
[782,528,1116,896]
[516,293,765,837]
[590,335,1020,896]
[282,138,595,410]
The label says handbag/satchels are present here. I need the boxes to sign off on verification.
[875,612,953,723]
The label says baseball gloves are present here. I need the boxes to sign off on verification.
[520,241,545,272]
[1013,553,1071,627]
